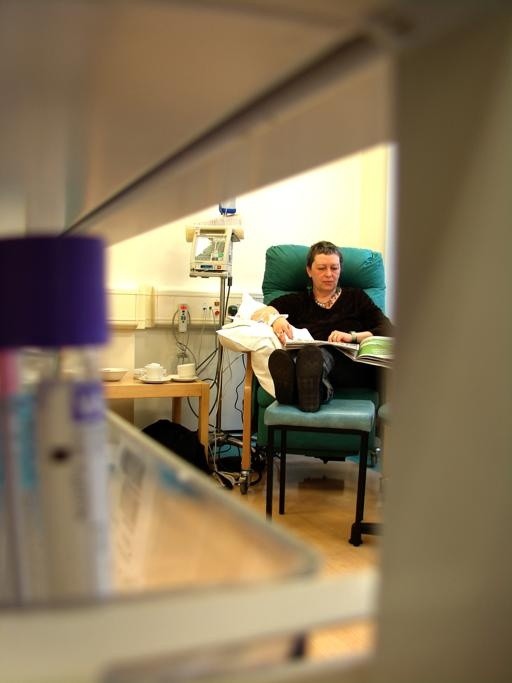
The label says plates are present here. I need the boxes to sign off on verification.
[139,374,200,384]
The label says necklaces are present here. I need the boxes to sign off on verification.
[314,286,342,309]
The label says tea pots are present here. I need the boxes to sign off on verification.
[144,363,165,378]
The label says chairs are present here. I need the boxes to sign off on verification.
[240,244,387,495]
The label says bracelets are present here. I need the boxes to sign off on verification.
[349,330,359,343]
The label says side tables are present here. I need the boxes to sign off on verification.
[102,374,210,468]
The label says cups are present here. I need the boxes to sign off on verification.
[176,363,196,377]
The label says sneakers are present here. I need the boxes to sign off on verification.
[297,344,324,414]
[268,349,296,406]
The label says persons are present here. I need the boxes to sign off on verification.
[250,241,396,414]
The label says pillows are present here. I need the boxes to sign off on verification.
[215,288,284,399]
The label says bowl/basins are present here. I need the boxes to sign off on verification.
[101,368,126,382]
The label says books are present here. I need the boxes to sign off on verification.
[280,334,360,351]
[332,336,395,371]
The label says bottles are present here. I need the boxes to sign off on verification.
[0,235,110,609]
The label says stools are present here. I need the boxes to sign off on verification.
[264,399,376,521]
